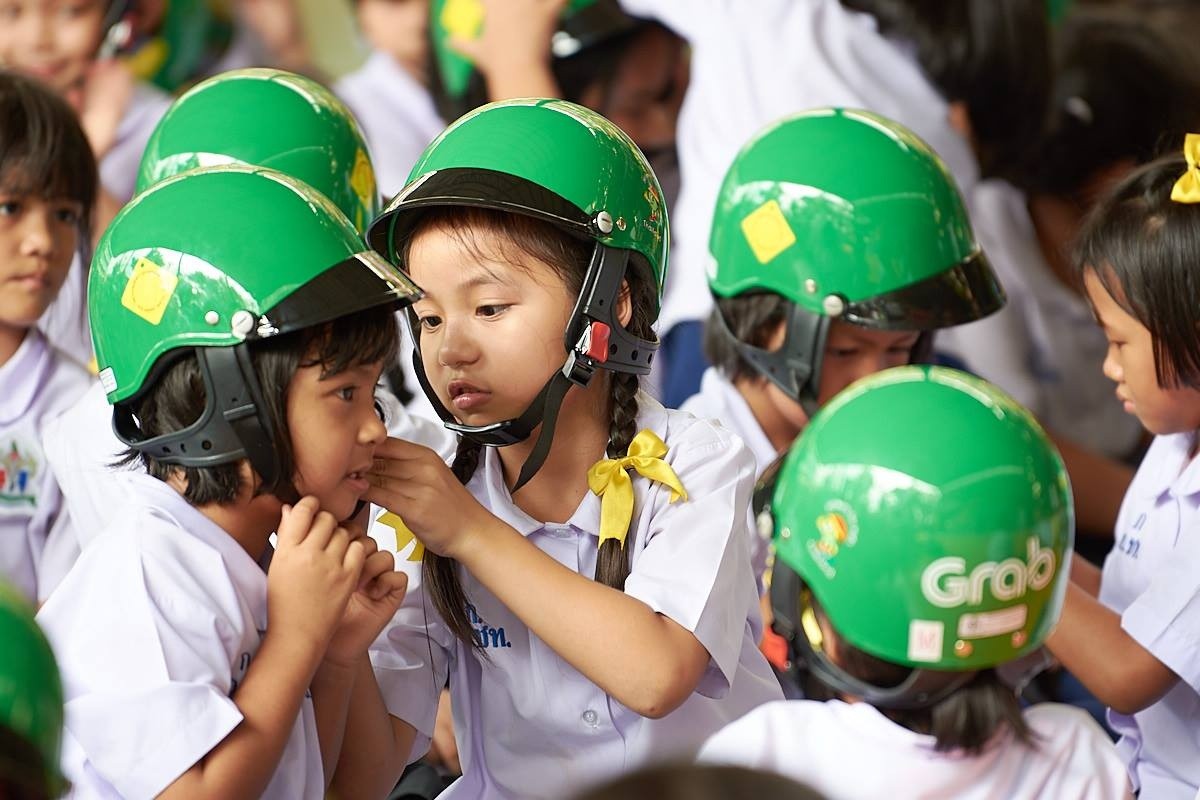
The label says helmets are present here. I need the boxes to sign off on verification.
[753,365,1074,670]
[703,107,1008,398]
[86,165,424,479]
[135,66,380,245]
[364,97,669,378]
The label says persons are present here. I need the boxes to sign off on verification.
[0,0,1200,800]
